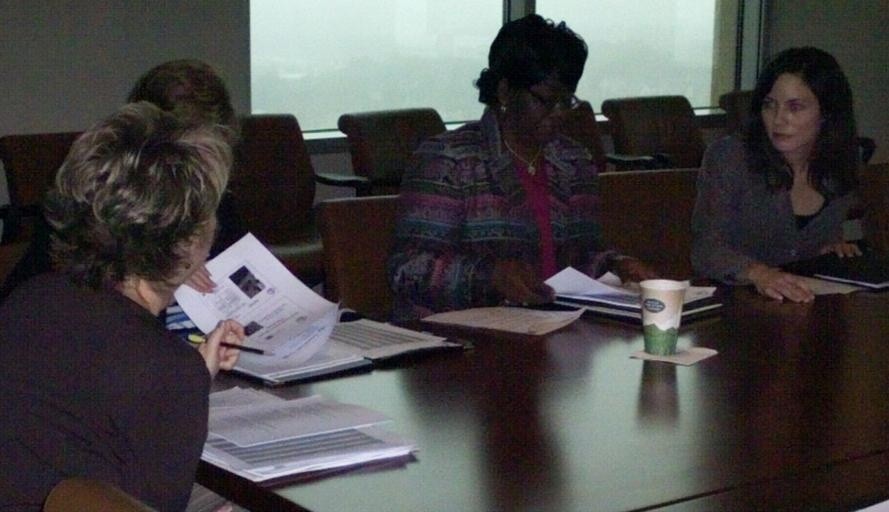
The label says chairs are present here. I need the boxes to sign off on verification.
[573,100,658,172]
[718,89,875,163]
[316,195,402,314]
[1,132,82,298]
[598,174,697,271]
[857,163,886,254]
[216,112,370,289]
[338,107,447,198]
[602,95,708,171]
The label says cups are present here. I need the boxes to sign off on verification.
[639,279,686,357]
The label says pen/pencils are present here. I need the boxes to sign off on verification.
[187,334,276,355]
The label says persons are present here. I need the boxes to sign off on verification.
[691,50,866,303]
[382,16,657,321]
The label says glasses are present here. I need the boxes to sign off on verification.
[528,87,580,111]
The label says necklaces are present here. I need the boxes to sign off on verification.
[503,141,545,175]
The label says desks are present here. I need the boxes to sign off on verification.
[155,256,887,510]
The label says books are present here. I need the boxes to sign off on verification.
[553,280,724,321]
[782,251,889,291]
